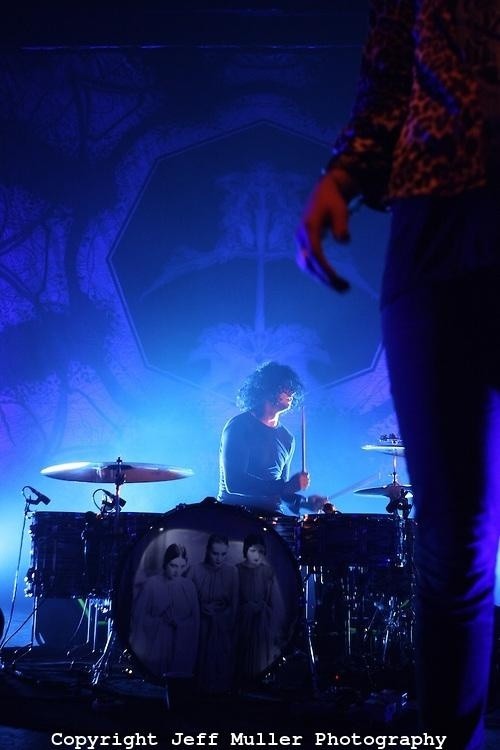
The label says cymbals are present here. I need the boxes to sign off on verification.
[41,460,190,485]
[362,441,405,451]
[355,482,413,498]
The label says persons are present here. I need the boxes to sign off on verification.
[294,3,499,750]
[128,532,286,688]
[217,362,329,517]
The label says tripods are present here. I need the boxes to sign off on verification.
[255,498,415,697]
[12,503,152,692]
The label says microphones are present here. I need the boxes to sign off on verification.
[32,488,53,505]
[103,491,126,507]
[386,491,409,512]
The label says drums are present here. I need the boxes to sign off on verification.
[365,514,404,559]
[228,502,303,563]
[310,512,367,556]
[94,512,165,599]
[29,511,99,599]
[111,497,312,690]
